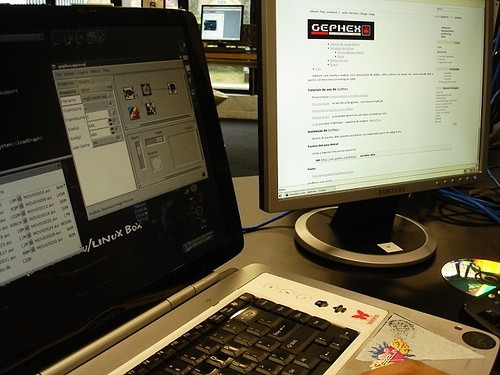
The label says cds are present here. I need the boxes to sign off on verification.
[440,257,500,297]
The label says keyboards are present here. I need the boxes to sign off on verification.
[204,47,245,54]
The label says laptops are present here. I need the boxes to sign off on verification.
[0,1,498,375]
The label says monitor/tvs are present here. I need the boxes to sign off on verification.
[200,5,243,43]
[249,0,489,214]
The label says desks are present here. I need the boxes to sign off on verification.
[217,175,499,375]
